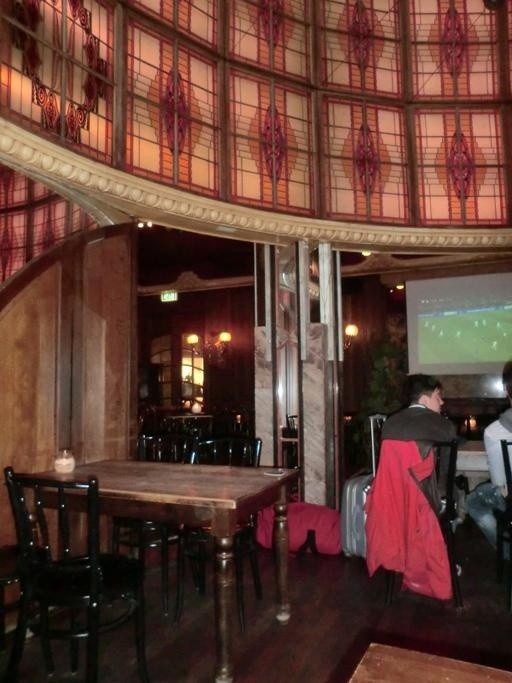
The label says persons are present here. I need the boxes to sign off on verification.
[464,361,511,585]
[380,371,463,579]
[416,294,512,350]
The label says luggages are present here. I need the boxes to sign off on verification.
[339,414,387,559]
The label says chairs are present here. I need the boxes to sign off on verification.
[0,411,512,634]
[2,466,150,683]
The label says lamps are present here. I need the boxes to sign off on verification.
[342,324,358,352]
[186,331,231,368]
